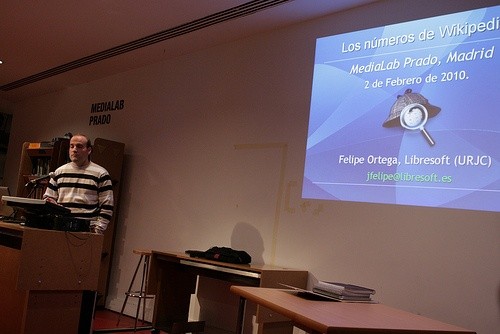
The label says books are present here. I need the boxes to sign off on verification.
[277,281,381,304]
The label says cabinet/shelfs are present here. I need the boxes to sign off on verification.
[15,137,126,312]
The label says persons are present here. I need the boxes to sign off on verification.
[42,134,113,234]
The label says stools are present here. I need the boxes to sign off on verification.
[116,250,156,333]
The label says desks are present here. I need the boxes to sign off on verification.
[147,249,478,334]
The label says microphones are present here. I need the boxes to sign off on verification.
[24,172,56,187]
